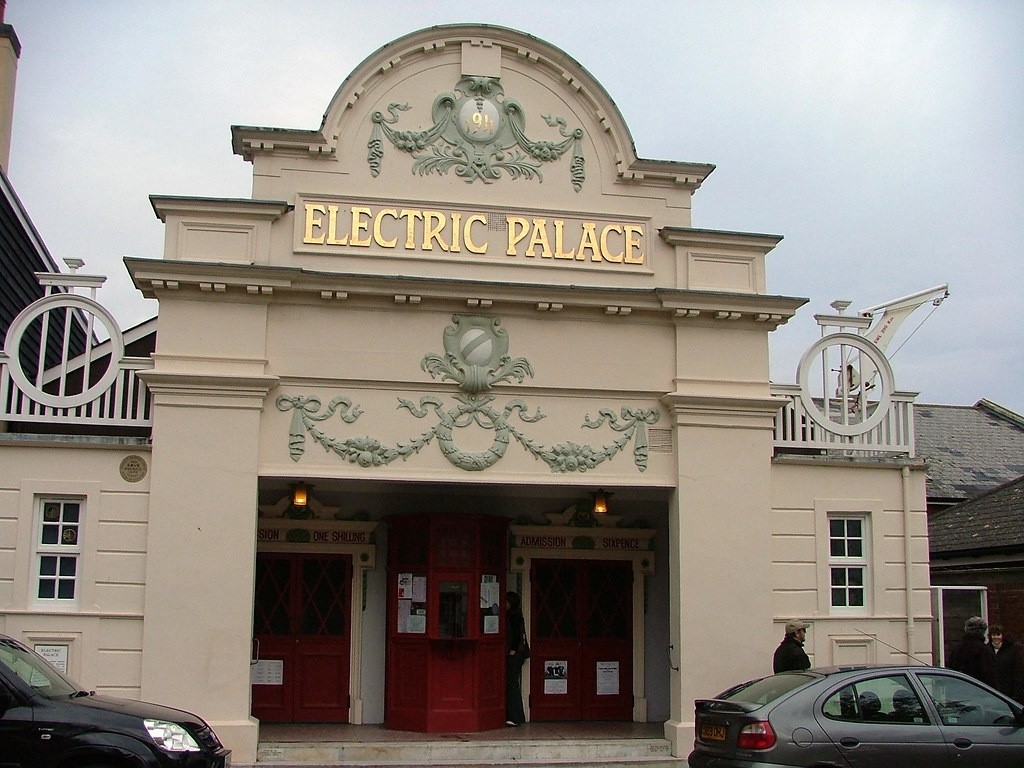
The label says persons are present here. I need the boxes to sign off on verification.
[504,591,530,728]
[545,661,566,676]
[773,617,811,675]
[945,616,1024,707]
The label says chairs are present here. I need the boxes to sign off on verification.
[888,689,926,722]
[859,692,888,721]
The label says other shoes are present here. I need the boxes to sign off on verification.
[506,720,518,726]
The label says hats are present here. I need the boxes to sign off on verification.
[785,619,811,634]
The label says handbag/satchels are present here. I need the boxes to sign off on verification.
[515,641,530,659]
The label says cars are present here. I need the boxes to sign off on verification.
[0,633,233,768]
[685,663,1024,768]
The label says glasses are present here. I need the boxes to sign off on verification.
[797,628,806,633]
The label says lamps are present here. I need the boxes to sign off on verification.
[287,481,315,509]
[588,488,614,513]
